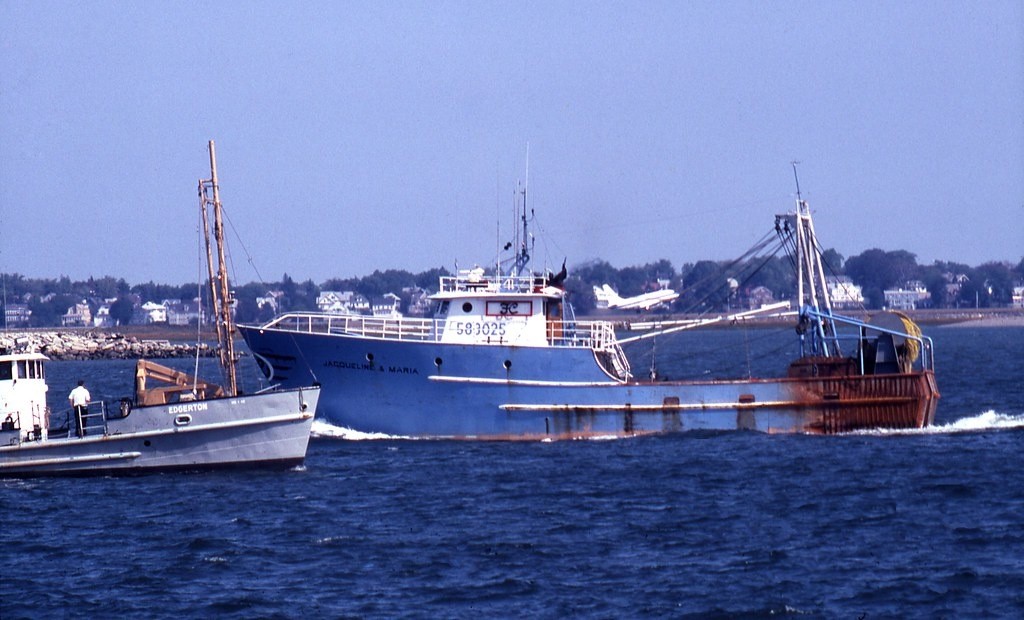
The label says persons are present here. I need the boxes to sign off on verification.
[69,380,91,437]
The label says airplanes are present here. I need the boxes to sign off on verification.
[593,284,680,311]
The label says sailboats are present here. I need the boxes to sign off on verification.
[1,139,323,468]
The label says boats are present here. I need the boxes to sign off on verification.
[0,336,51,439]
[214,139,940,436]
